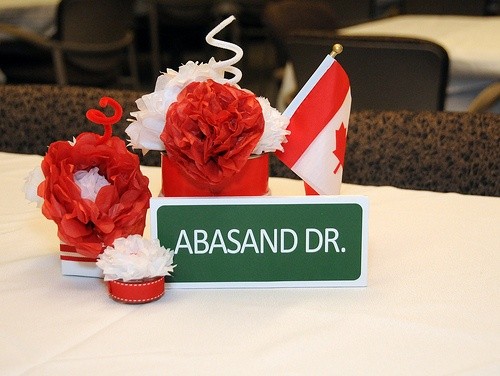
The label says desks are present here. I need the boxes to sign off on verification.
[337,15,500,112]
[1,151,500,375]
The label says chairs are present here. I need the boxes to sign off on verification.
[0,84,162,166]
[270,113,500,197]
[8,0,488,112]
